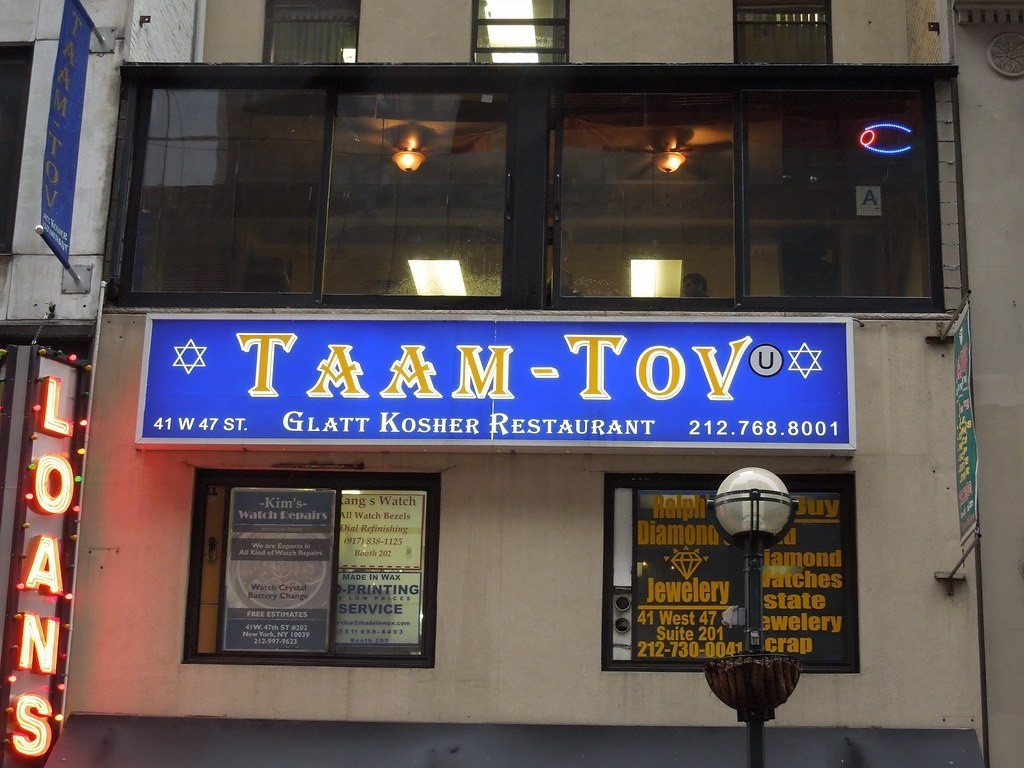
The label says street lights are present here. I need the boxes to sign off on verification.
[707,466,803,768]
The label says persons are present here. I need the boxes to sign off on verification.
[561,271,577,295]
[810,244,839,295]
[682,274,707,297]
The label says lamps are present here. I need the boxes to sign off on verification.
[653,153,687,175]
[391,151,427,172]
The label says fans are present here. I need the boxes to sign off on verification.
[605,128,733,176]
